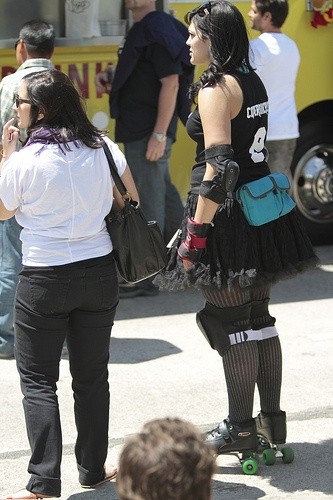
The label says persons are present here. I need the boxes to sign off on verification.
[0,70,140,500]
[248,0,300,198]
[95,0,196,298]
[152,0,320,475]
[0,21,74,358]
[116,416,217,500]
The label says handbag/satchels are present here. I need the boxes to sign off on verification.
[105,196,170,285]
[233,171,296,227]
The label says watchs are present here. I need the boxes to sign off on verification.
[151,132,167,142]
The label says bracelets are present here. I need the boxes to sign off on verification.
[0,149,7,158]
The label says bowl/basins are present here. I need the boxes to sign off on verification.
[99,20,127,36]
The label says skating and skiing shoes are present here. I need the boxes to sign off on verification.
[253,410,295,466]
[200,417,262,474]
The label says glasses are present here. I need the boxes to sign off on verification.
[198,0,212,18]
[10,92,32,108]
[14,39,20,51]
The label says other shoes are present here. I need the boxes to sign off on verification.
[80,463,118,488]
[0,486,62,499]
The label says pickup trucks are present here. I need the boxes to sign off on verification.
[0,1,333,245]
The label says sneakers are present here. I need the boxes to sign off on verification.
[117,280,161,299]
